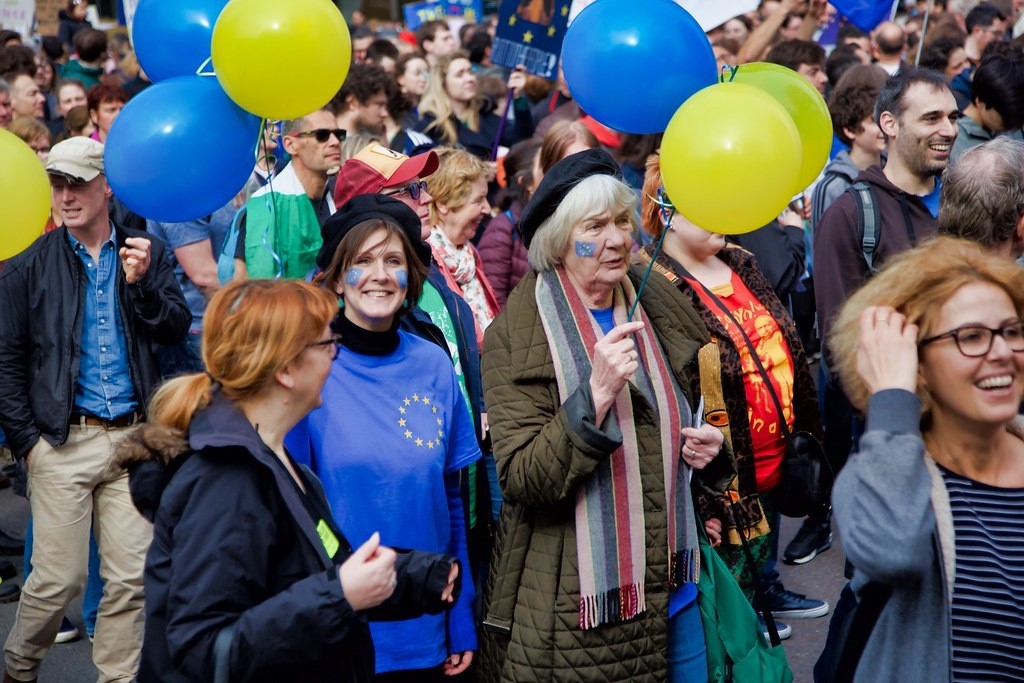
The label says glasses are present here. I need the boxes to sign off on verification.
[383,181,427,199]
[287,128,347,143]
[302,333,343,360]
[916,320,1024,356]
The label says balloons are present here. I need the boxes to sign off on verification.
[213,0,354,123]
[661,82,805,235]
[722,62,834,196]
[103,78,262,223]
[0,126,54,262]
[132,0,229,85]
[561,0,718,136]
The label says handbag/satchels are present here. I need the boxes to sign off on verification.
[779,430,829,517]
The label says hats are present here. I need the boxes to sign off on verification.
[316,193,431,298]
[519,148,622,250]
[46,136,107,184]
[333,142,438,209]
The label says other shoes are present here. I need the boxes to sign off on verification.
[0,583,21,602]
[0,560,16,577]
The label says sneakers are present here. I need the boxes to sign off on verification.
[753,581,829,618]
[783,520,829,564]
[53,615,79,643]
[759,620,790,641]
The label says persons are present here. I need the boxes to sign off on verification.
[0,0,1024,683]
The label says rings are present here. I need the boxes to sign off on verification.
[627,354,633,360]
[875,317,890,325]
[691,450,695,460]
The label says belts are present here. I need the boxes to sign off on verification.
[67,412,148,432]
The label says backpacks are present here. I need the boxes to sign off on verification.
[691,536,791,683]
